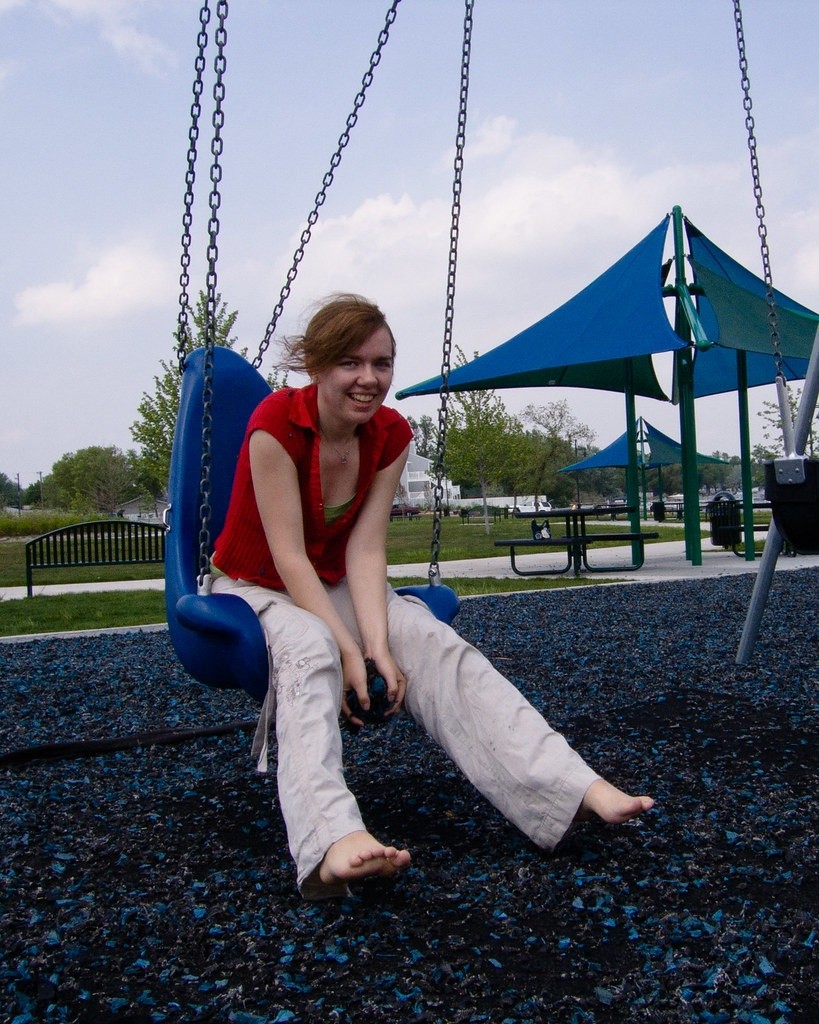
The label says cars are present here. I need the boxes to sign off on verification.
[390,503,420,516]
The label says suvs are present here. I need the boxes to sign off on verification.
[507,500,552,515]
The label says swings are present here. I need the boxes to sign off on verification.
[718,0,819,558]
[163,0,481,704]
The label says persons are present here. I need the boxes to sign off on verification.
[208,298,654,898]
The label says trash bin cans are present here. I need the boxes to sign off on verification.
[705,493,743,546]
[653,501,665,522]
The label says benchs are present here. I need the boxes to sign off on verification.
[718,525,769,557]
[560,532,659,572]
[492,512,514,522]
[665,509,685,519]
[494,538,593,577]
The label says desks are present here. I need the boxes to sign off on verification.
[732,503,773,508]
[492,507,515,519]
[664,502,683,505]
[515,506,638,574]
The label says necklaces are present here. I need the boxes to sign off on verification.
[320,427,354,463]
